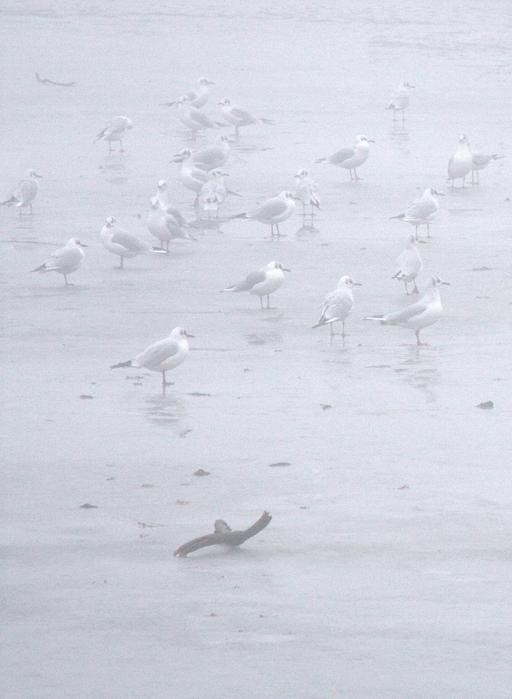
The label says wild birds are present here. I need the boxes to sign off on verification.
[0,169,43,216]
[31,236,88,286]
[445,134,504,189]
[314,132,377,182]
[111,325,194,397]
[32,71,78,96]
[221,262,292,309]
[87,74,321,273]
[389,187,445,243]
[391,234,420,298]
[314,274,449,346]
[385,80,417,135]
